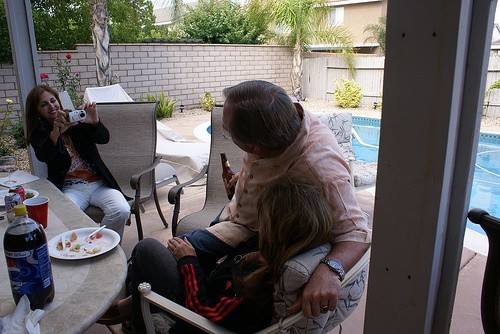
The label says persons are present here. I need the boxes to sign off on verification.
[25,86,130,243]
[96,81,371,334]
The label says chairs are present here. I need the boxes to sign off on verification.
[84,101,168,241]
[138,105,379,334]
[467,208,500,334]
[83,84,209,186]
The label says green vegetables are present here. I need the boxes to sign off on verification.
[25,192,34,199]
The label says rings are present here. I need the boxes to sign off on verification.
[321,306,328,312]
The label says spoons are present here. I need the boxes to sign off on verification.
[77,225,106,245]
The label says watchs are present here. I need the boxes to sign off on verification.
[321,258,346,282]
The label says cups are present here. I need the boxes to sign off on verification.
[23,197,49,229]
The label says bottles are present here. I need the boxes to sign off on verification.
[221,153,237,200]
[3,204,55,312]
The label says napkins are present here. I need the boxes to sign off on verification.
[0,294,45,334]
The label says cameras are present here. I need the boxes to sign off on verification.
[67,109,87,123]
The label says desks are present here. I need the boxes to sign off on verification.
[0,172,128,333]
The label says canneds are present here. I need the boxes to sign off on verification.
[4,192,22,223]
[8,185,25,203]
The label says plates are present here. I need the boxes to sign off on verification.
[0,189,39,206]
[48,227,120,260]
